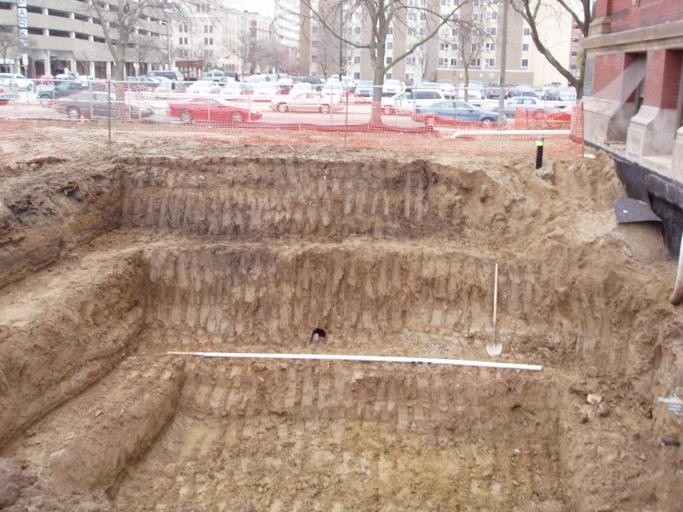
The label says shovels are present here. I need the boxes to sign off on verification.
[486,263,503,357]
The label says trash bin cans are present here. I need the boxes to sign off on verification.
[514,108,528,130]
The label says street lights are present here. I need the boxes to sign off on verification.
[158,8,175,71]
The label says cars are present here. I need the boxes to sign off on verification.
[489,95,546,119]
[168,95,263,124]
[269,89,345,114]
[52,90,155,124]
[480,81,578,102]
[410,99,507,129]
[380,87,446,116]
[184,69,483,105]
[0,67,186,109]
